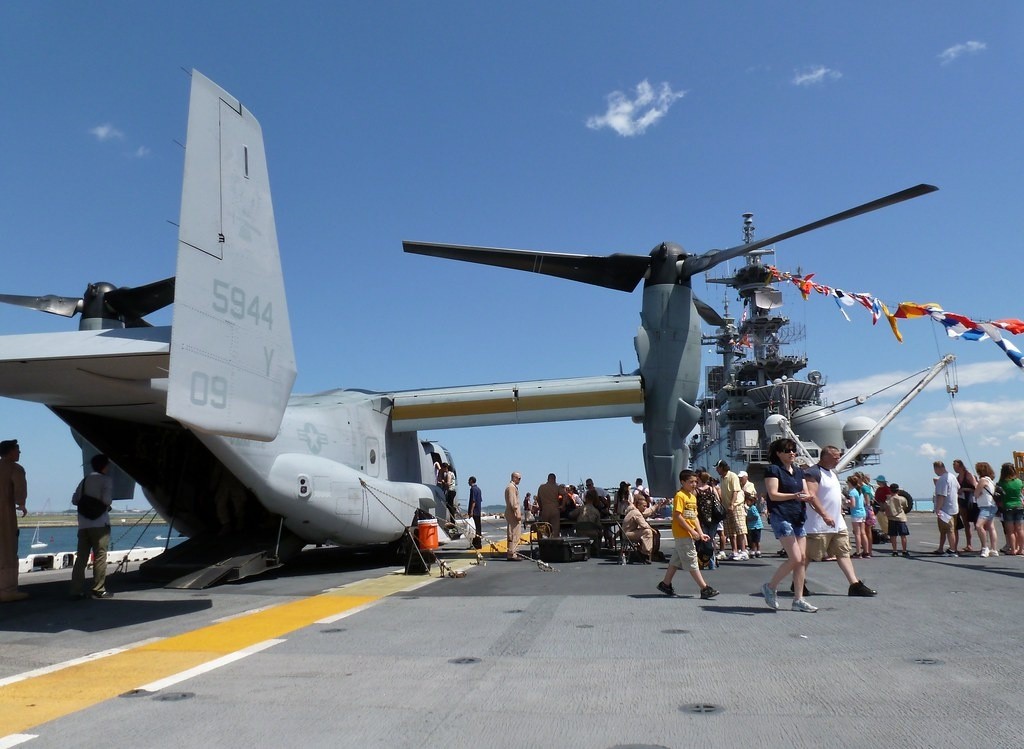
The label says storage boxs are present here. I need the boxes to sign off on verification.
[540,536,591,563]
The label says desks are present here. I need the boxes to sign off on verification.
[523,519,622,529]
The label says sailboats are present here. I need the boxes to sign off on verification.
[30,521,49,549]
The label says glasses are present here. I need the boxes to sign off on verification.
[783,448,797,454]
[518,478,521,480]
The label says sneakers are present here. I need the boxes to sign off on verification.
[700,585,720,598]
[3,593,30,602]
[74,590,114,599]
[792,598,818,613]
[656,581,677,598]
[762,584,779,609]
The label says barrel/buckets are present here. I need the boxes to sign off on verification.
[417,519,439,550]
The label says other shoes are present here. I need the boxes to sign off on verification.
[642,557,653,565]
[892,551,898,557]
[861,553,871,558]
[902,552,910,558]
[470,546,482,549]
[848,581,877,596]
[850,552,862,559]
[934,544,1024,557]
[507,556,525,561]
[715,540,761,560]
[791,580,813,595]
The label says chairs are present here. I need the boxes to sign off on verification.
[615,521,646,565]
[530,522,553,559]
[575,522,603,557]
[405,527,442,575]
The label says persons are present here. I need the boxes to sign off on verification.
[760,439,877,611]
[0,439,29,602]
[70,454,113,599]
[656,470,720,599]
[873,474,911,558]
[468,476,482,549]
[692,459,764,569]
[432,456,457,529]
[931,459,1024,556]
[613,478,672,560]
[524,472,616,554]
[504,472,523,561]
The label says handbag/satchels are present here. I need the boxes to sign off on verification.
[956,514,965,530]
[77,496,107,519]
[465,517,476,538]
[715,498,725,523]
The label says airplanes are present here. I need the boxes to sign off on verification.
[0,68,940,592]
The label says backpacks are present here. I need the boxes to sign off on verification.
[984,478,1005,503]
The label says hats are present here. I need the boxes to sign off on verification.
[713,458,728,467]
[695,466,707,474]
[873,475,888,482]
[738,471,749,477]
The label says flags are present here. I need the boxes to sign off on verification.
[772,269,1024,369]
